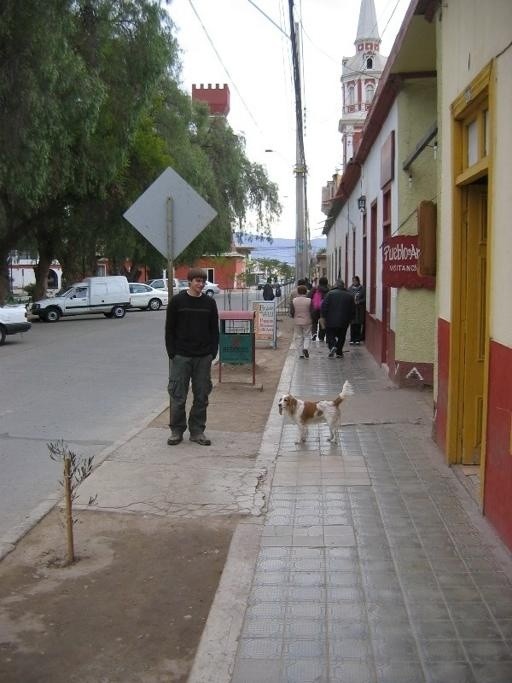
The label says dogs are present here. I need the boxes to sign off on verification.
[278,379,354,446]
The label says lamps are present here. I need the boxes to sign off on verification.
[408,174,413,189]
[433,134,438,162]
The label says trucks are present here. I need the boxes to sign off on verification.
[32,275,132,322]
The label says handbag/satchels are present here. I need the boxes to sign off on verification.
[312,291,322,310]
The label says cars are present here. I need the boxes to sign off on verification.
[144,278,188,299]
[0,304,32,346]
[257,276,294,290]
[179,279,220,298]
[127,282,169,311]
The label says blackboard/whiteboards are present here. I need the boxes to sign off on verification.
[251,301,277,340]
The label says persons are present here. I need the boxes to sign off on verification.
[289,275,366,360]
[164,266,217,446]
[262,276,275,300]
[276,284,281,297]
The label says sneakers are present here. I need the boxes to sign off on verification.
[350,341,359,345]
[312,334,324,342]
[328,347,344,358]
[167,430,183,445]
[190,434,211,446]
[300,349,309,358]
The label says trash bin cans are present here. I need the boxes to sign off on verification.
[218,310,256,366]
